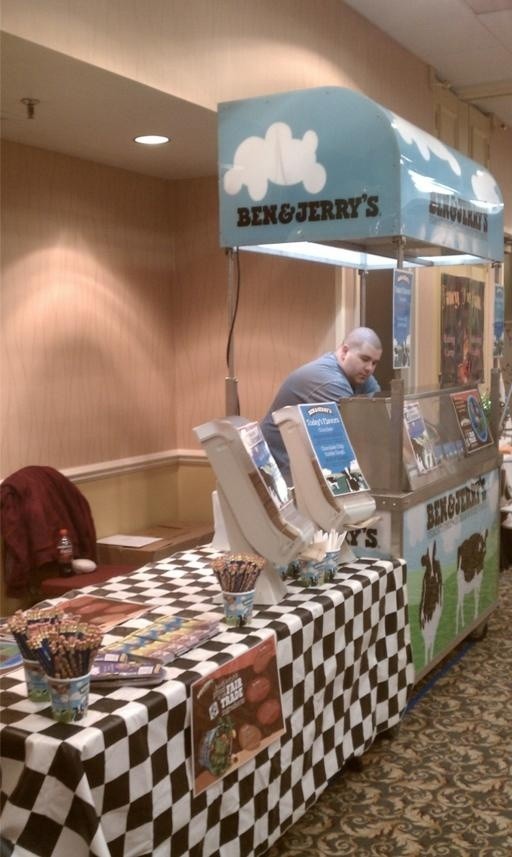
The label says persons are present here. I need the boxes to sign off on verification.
[257,327,383,488]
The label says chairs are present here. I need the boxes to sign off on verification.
[0,465,142,603]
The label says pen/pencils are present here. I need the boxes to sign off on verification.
[213,554,263,591]
[8,606,104,678]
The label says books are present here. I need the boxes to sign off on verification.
[90,614,221,687]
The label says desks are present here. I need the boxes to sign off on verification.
[0,544,406,857]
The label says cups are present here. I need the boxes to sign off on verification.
[299,549,339,588]
[22,658,91,722]
[222,590,254,626]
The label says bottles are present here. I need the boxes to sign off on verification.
[55,529,75,577]
